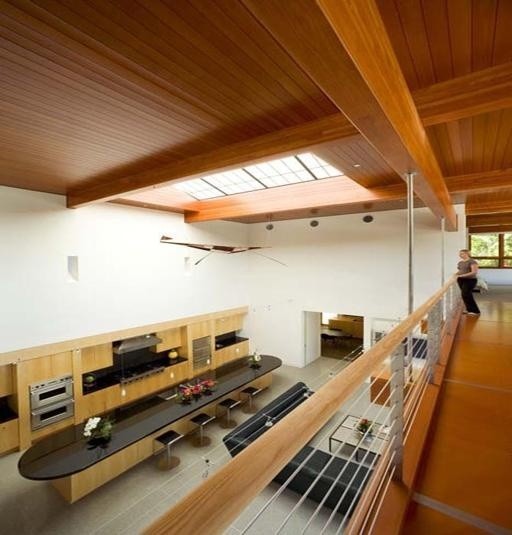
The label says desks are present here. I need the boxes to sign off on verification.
[18,355,283,505]
[370,367,424,408]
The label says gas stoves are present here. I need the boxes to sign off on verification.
[111,362,165,388]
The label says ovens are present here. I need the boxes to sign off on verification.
[28,373,75,432]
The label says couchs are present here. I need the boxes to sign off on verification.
[221,381,376,518]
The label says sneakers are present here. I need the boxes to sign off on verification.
[462,306,479,317]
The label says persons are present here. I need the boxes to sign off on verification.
[454,249,481,316]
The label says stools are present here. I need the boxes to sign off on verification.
[240,386,262,414]
[216,399,241,429]
[189,412,215,447]
[152,430,184,470]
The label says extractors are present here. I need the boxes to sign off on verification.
[112,334,163,355]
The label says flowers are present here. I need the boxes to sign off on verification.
[187,380,202,395]
[248,350,261,363]
[201,378,216,393]
[84,415,115,440]
[355,418,372,432]
[177,384,192,402]
